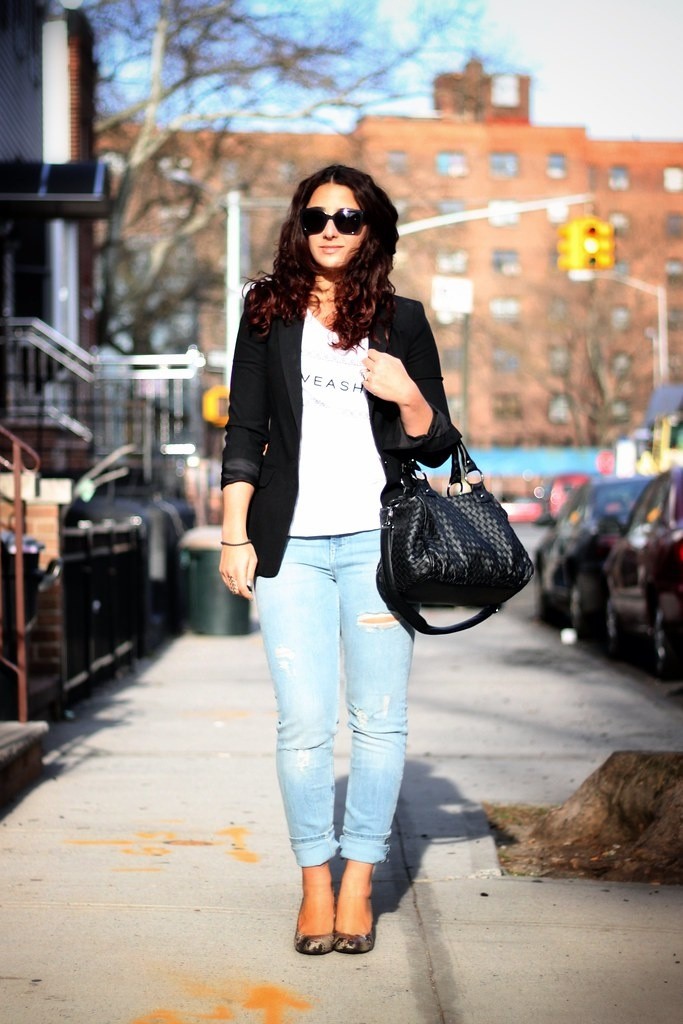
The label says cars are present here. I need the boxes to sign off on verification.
[530,474,655,642]
[598,468,682,679]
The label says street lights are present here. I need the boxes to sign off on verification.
[567,269,670,394]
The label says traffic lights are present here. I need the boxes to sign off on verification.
[569,218,613,270]
[557,224,573,270]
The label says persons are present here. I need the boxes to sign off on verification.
[214,162,461,959]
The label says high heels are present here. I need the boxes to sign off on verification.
[335,890,376,955]
[294,889,334,955]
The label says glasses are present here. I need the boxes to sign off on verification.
[297,205,369,235]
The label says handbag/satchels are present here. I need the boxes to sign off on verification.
[376,401,535,635]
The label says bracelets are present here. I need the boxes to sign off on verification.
[221,539,252,547]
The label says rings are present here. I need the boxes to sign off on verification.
[361,371,373,381]
[229,574,239,595]
